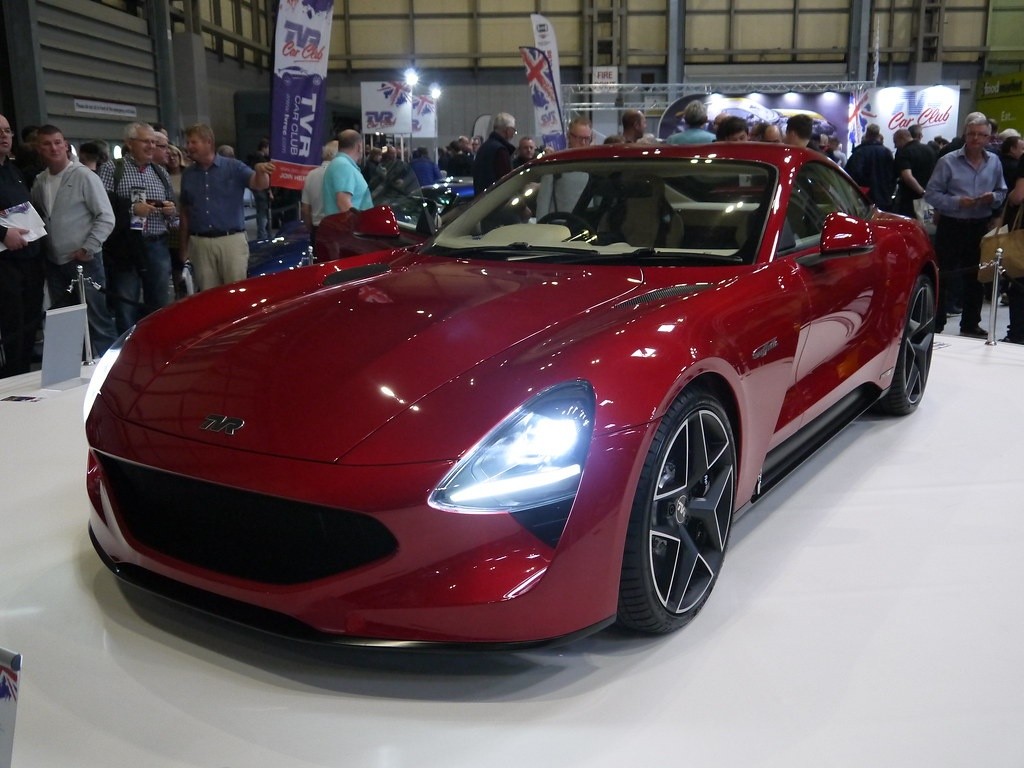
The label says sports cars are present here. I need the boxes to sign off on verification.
[77,141,941,649]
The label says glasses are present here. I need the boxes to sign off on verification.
[128,138,156,145]
[570,132,591,142]
[154,145,170,150]
[0,129,14,137]
[964,132,990,138]
[507,126,518,135]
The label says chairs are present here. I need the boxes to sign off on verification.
[715,186,812,249]
[596,175,684,249]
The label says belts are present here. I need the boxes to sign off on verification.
[190,229,245,238]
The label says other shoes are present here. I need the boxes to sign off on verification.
[960,326,989,339]
[947,306,963,314]
[1000,293,1009,306]
[1007,325,1011,329]
[997,336,1024,345]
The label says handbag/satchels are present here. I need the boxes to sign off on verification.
[913,197,937,236]
[977,191,1024,282]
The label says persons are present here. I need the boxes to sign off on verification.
[1,57,1024,371]
